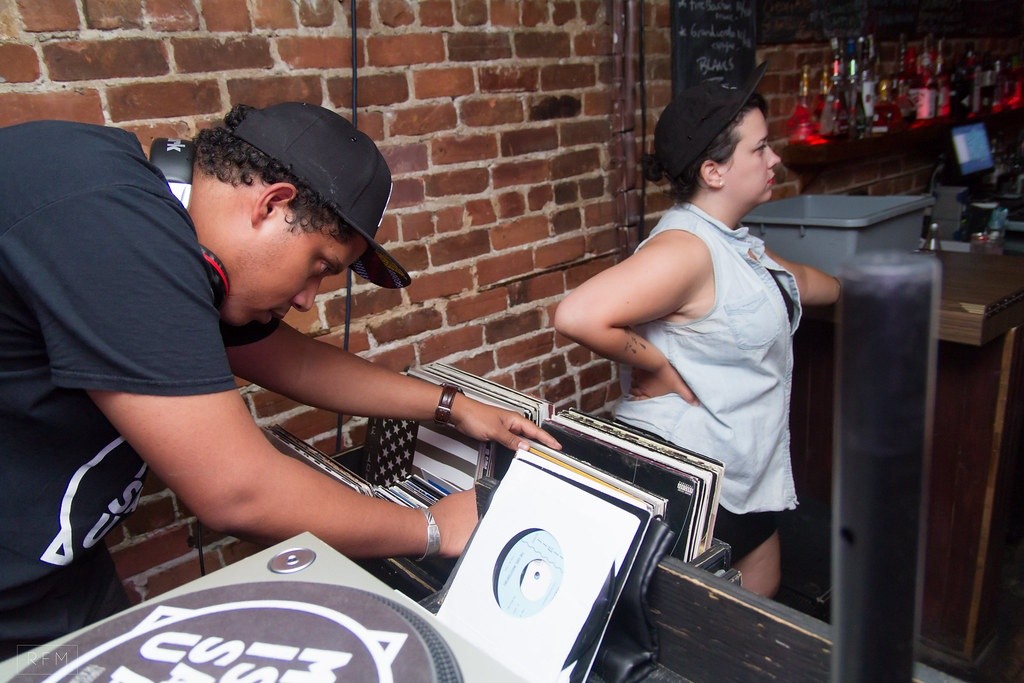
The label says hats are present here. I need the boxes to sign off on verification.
[653,60,769,183]
[223,101,412,290]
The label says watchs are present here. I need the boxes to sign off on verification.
[433,382,465,425]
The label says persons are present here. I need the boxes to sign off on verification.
[554,81,849,605]
[1,102,548,662]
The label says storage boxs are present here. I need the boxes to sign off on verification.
[331,442,746,655]
[734,193,935,283]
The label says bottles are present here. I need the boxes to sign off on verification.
[783,30,1024,143]
[924,218,943,252]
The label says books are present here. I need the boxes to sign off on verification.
[377,469,464,508]
[434,438,670,683]
[261,423,376,498]
[542,406,724,564]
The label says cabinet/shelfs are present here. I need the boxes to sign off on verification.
[771,103,1022,167]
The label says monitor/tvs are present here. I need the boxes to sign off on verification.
[946,120,995,180]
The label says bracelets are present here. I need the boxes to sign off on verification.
[831,276,842,307]
[413,506,442,562]
